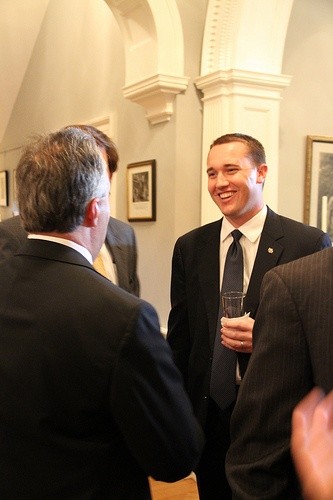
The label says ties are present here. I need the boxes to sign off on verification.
[209,229,244,411]
[93,252,106,276]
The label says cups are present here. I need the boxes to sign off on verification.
[221,291,246,318]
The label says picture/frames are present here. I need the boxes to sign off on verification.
[0,168,19,218]
[303,134,333,241]
[126,159,157,222]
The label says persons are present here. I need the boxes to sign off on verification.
[165,133,328,500]
[1,130,208,500]
[0,124,140,301]
[226,194,333,500]
